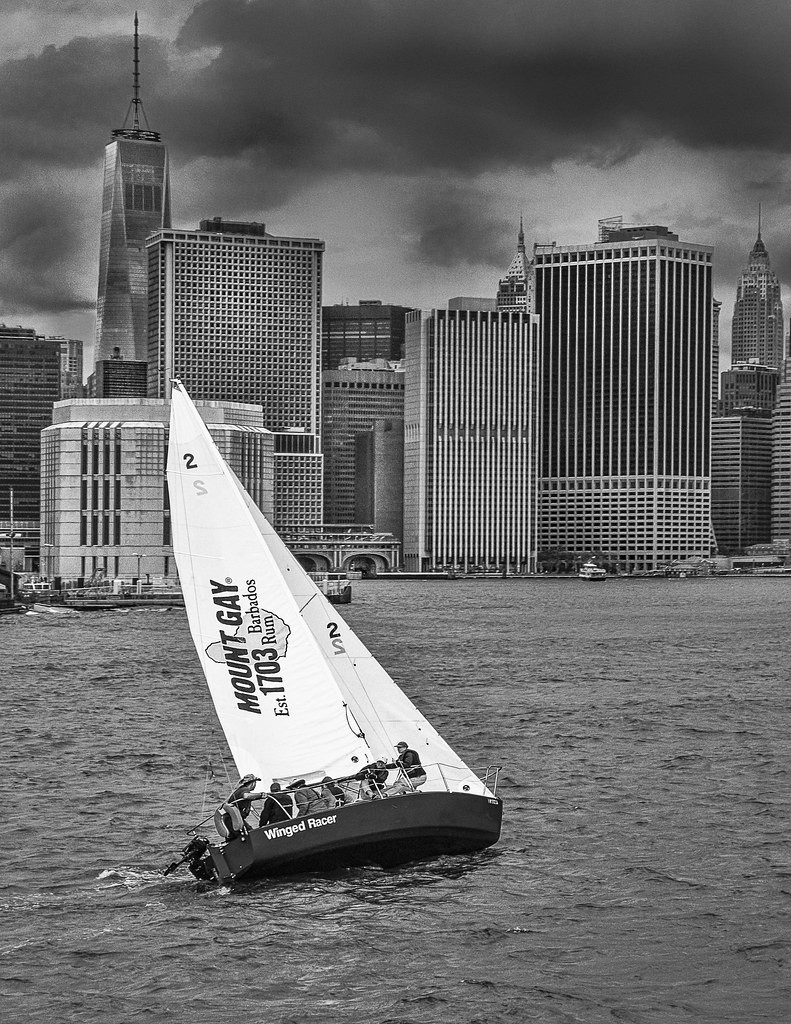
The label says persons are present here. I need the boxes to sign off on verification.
[321,777,346,807]
[259,783,293,827]
[287,779,326,818]
[359,758,389,800]
[222,774,267,838]
[382,741,427,797]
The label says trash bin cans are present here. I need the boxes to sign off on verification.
[132,578,138,586]
[77,578,85,588]
[55,575,61,595]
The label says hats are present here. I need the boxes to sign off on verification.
[239,773,261,784]
[377,757,388,763]
[286,778,305,788]
[393,742,408,747]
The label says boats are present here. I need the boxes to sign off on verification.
[579,563,607,582]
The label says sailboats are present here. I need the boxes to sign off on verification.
[165,376,506,887]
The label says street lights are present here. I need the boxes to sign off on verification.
[132,553,147,580]
[0,533,22,601]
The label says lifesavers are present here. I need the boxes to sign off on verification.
[213,804,243,838]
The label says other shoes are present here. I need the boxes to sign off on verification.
[370,794,376,799]
